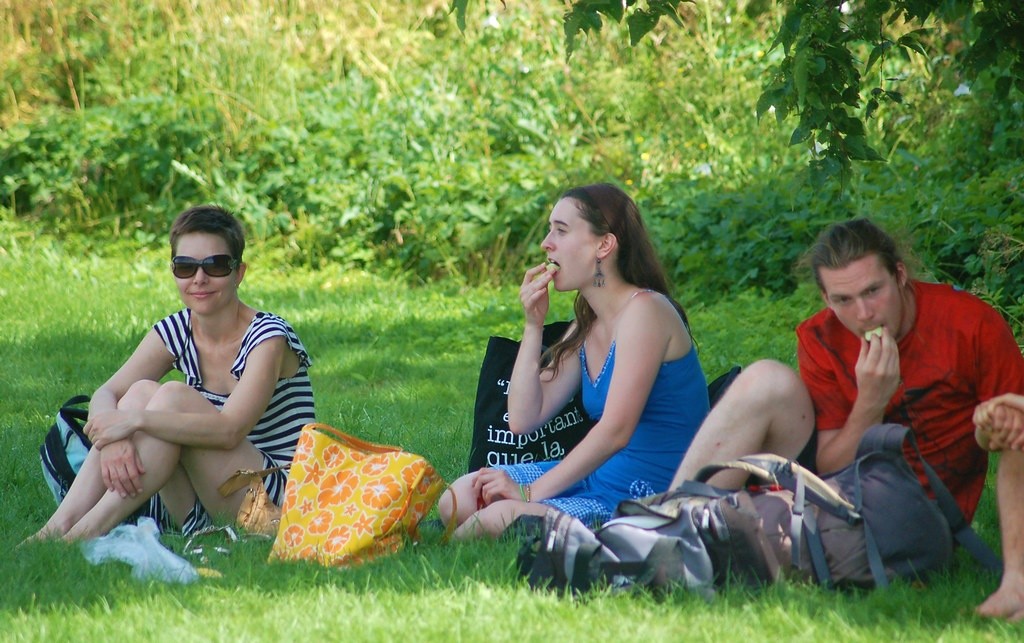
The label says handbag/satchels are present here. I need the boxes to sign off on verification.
[259,423,459,569]
[465,336,595,472]
[516,504,741,600]
[216,462,294,543]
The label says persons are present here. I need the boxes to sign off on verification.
[973,392,1024,622]
[8,204,315,549]
[666,218,1024,589]
[438,183,712,548]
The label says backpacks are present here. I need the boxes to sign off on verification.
[39,394,99,504]
[701,364,746,417]
[613,419,1007,596]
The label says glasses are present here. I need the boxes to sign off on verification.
[171,254,238,280]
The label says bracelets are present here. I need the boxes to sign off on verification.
[519,484,526,501]
[526,484,530,502]
